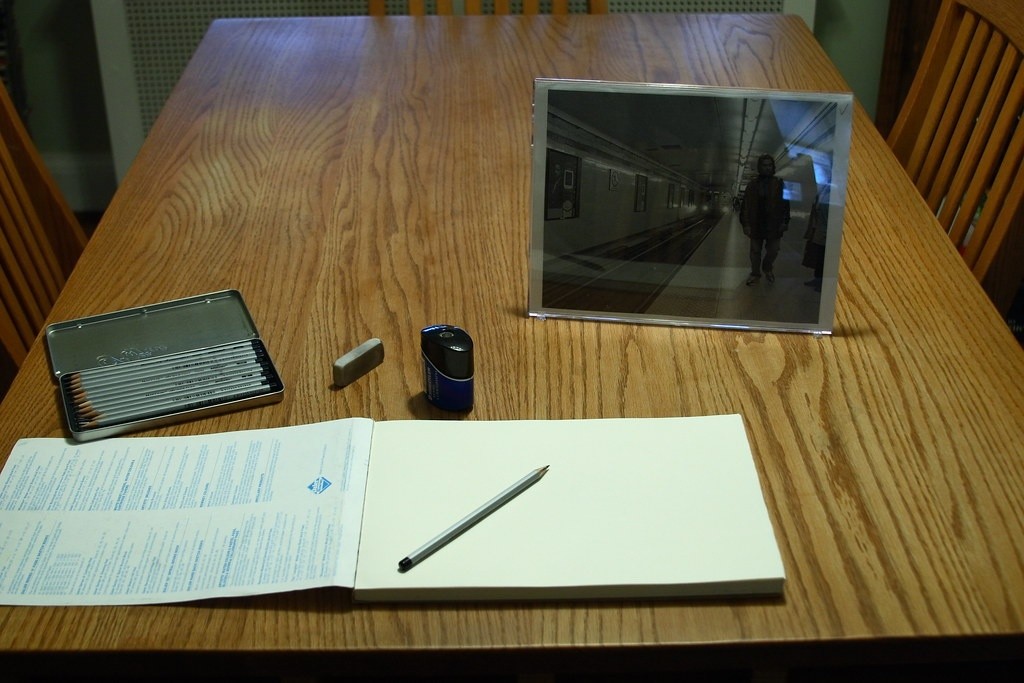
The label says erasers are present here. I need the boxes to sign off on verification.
[332,337,385,386]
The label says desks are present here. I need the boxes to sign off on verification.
[0,11,1024,683]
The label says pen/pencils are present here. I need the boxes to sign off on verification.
[397,464,550,572]
[61,340,279,428]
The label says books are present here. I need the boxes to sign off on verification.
[0,414,787,606]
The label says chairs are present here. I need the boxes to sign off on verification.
[0,80,86,371]
[883,0,1024,323]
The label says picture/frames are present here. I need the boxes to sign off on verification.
[527,76,853,338]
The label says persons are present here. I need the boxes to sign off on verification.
[800,152,833,292]
[549,161,567,201]
[739,153,790,285]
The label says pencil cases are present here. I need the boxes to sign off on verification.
[45,288,286,442]
